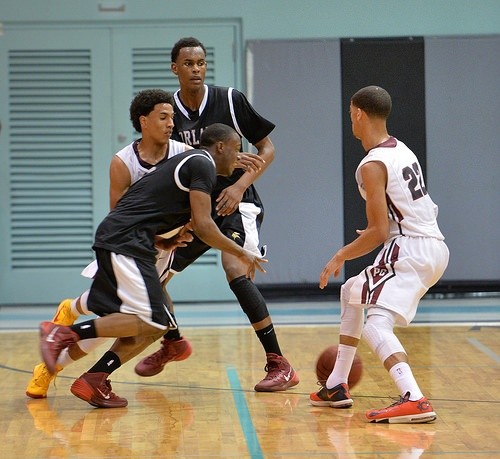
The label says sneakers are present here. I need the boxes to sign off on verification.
[38,321,79,375]
[26,361,63,399]
[253,354,299,393]
[309,405,353,433]
[310,379,354,408]
[71,372,128,408]
[366,421,437,450]
[51,299,77,326]
[365,391,437,426]
[26,396,63,434]
[255,388,300,418]
[135,337,192,377]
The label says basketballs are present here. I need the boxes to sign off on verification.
[316,345,363,390]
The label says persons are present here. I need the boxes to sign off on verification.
[26,90,266,397]
[41,123,269,408]
[309,85,450,423]
[135,36,300,393]
